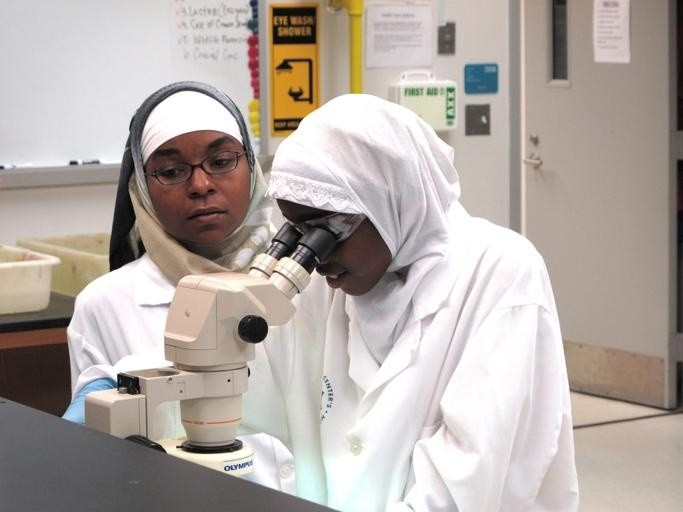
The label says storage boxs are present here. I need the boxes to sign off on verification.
[21,232,116,301]
[0,235,64,316]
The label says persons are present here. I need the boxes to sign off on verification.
[62,93,580,512]
[66,79,283,398]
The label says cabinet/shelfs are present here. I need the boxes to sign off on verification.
[0,289,341,512]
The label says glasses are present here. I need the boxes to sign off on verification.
[280,213,367,245]
[143,150,248,185]
